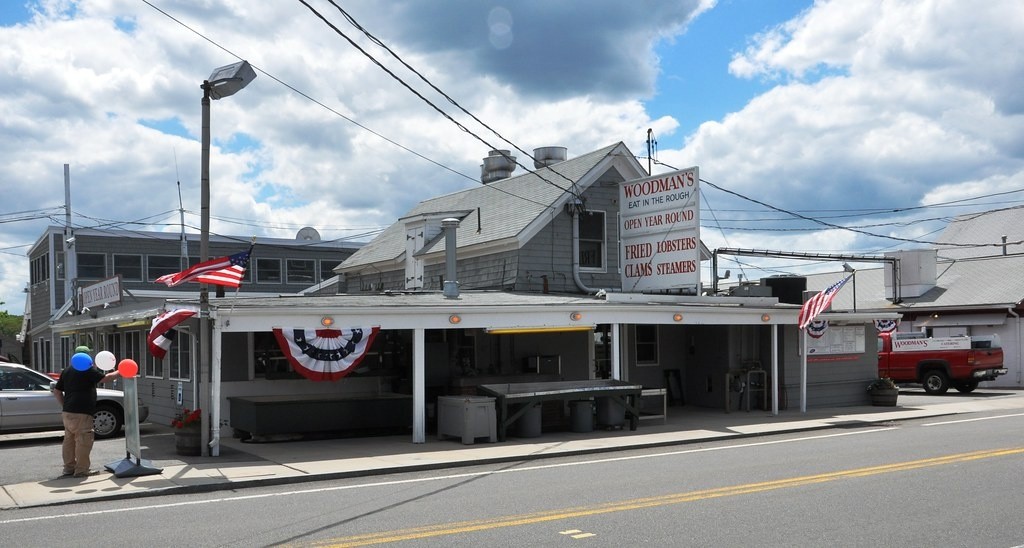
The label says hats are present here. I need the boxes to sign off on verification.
[75,346,94,354]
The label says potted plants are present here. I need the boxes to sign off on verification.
[864,377,899,407]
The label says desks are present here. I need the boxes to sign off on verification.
[626,388,668,422]
[480,377,643,443]
[226,392,429,443]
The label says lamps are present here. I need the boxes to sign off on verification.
[81,306,90,314]
[673,313,683,321]
[570,312,583,321]
[321,316,334,326]
[929,312,939,319]
[448,314,461,324]
[68,308,76,316]
[761,313,770,322]
[103,303,113,310]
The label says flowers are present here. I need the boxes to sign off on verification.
[169,406,211,428]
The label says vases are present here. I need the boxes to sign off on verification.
[174,426,213,457]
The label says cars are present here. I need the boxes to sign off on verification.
[0,362,149,439]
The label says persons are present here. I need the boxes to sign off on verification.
[55,345,122,478]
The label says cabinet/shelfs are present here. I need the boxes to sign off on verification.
[436,395,497,446]
[724,368,768,413]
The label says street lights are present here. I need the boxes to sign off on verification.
[198,59,258,459]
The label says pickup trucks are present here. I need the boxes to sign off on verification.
[877,332,1009,396]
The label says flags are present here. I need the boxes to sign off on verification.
[798,275,852,330]
[153,245,251,287]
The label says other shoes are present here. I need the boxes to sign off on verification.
[63,468,74,474]
[73,468,99,478]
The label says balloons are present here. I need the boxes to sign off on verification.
[95,351,116,370]
[71,353,93,371]
[118,359,138,378]
